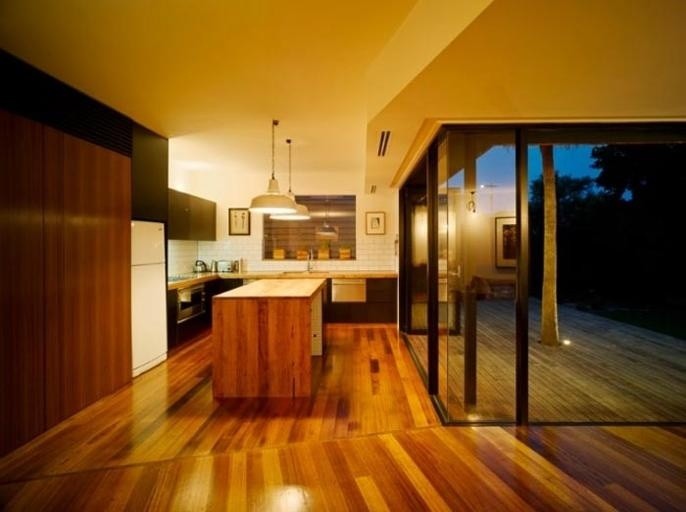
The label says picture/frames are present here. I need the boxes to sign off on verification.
[229,209,250,236]
[365,212,385,235]
[495,217,517,269]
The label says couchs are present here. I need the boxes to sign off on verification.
[470,276,516,300]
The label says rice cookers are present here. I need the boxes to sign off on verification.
[216,260,231,272]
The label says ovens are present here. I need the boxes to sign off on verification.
[176,283,204,323]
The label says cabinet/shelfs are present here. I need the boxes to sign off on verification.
[168,188,215,240]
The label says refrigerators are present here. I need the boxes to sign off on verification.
[130,218,168,379]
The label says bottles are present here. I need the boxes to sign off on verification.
[210,261,215,271]
[233,260,238,271]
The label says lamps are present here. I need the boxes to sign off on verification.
[249,120,299,214]
[269,140,310,220]
[315,195,337,235]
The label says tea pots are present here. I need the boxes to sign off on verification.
[192,260,205,272]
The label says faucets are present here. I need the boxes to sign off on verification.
[310,250,314,261]
[305,254,312,271]
